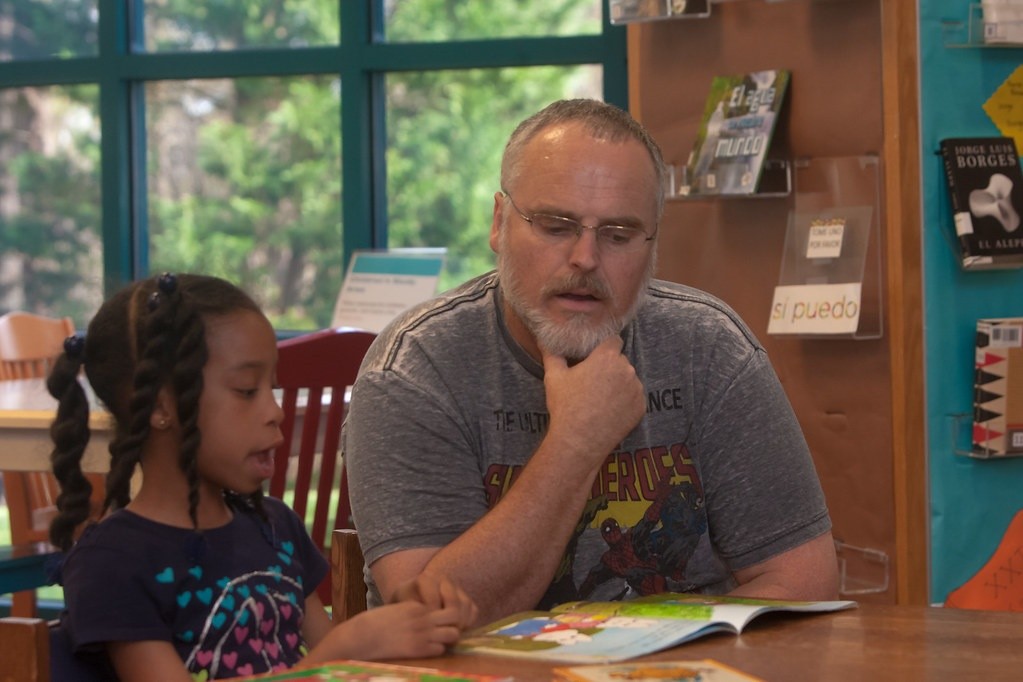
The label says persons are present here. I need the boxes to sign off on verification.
[940,137,1023,270]
[981,0,1023,48]
[49,276,478,682]
[344,98,839,637]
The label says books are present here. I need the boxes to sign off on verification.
[972,316,1023,459]
[555,661,765,682]
[684,69,794,194]
[454,591,858,665]
[221,660,515,682]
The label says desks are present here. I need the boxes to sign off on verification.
[374,606,1023,682]
[0,389,353,474]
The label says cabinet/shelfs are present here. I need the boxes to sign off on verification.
[607,2,1022,596]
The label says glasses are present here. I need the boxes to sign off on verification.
[502,190,660,254]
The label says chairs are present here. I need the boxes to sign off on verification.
[0,310,85,615]
[267,326,377,608]
